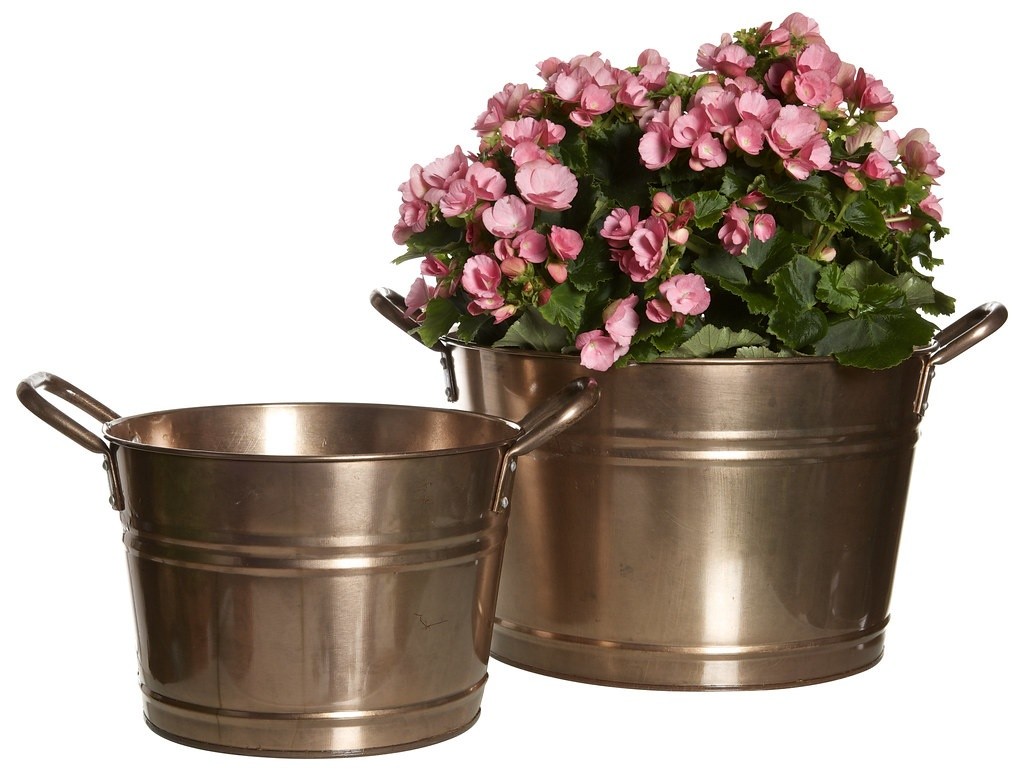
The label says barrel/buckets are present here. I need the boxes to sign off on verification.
[19,371,602,756]
[368,282,1008,691]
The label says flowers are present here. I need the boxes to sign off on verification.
[393,12,956,372]
